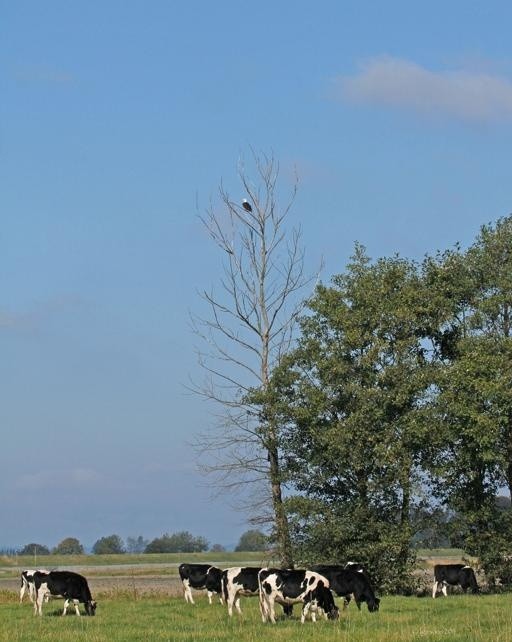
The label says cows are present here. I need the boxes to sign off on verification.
[18,568,64,603]
[31,569,97,618]
[177,562,229,605]
[431,563,480,599]
[318,568,381,613]
[255,567,340,626]
[219,566,295,618]
